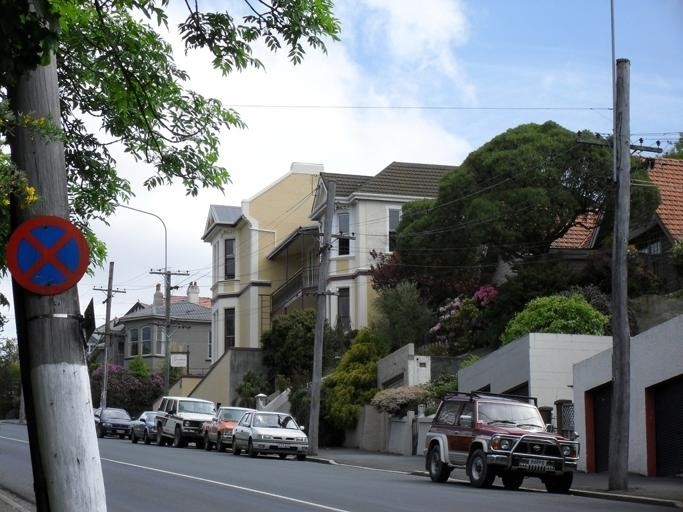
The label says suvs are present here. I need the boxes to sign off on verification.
[424,390,580,494]
[155,396,219,449]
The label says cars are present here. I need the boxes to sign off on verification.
[92,408,174,446]
[200,407,309,461]
